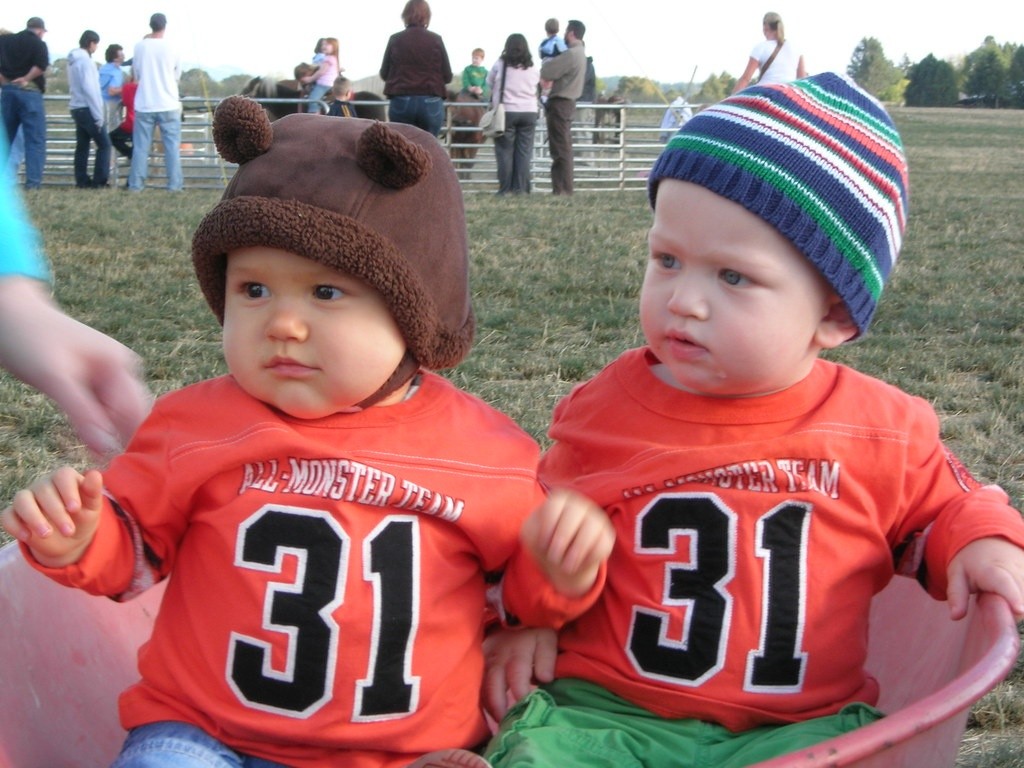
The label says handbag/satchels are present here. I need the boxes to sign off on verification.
[478,105,505,138]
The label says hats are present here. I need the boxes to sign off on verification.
[27,17,48,32]
[651,69,912,341]
[192,96,476,368]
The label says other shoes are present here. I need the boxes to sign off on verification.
[77,181,94,188]
[538,99,549,109]
[92,182,110,189]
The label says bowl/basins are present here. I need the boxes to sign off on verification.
[1,532,1017,767]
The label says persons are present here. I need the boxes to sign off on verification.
[126,12,183,191]
[538,18,568,107]
[479,73,1024,768]
[0,93,615,768]
[485,33,540,194]
[539,20,587,195]
[731,12,807,95]
[294,36,358,117]
[66,29,111,189]
[109,63,138,160]
[0,16,49,186]
[460,48,489,100]
[99,44,126,130]
[379,0,453,138]
[0,117,150,456]
[573,55,596,165]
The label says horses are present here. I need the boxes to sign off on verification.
[444,89,488,179]
[238,75,386,121]
